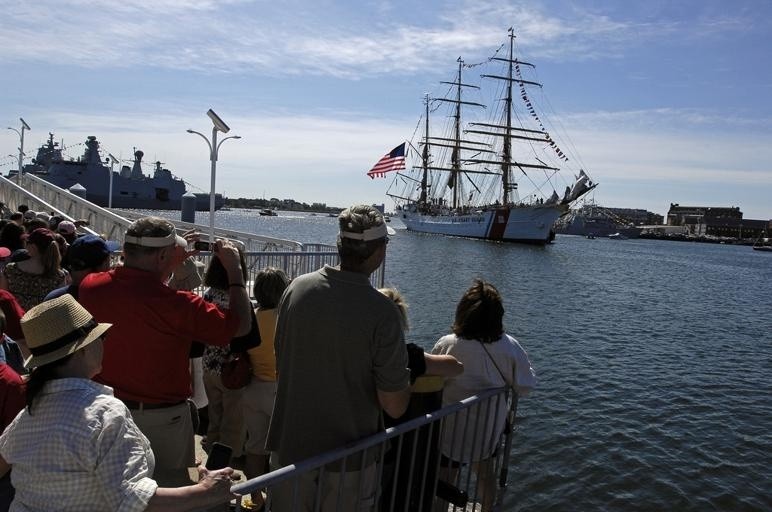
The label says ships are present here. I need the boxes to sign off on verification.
[553,198,643,239]
[23,130,232,212]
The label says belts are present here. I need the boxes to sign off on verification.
[120,399,186,411]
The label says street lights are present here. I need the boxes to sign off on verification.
[9,154,20,165]
[98,153,120,209]
[7,117,31,187]
[186,108,243,244]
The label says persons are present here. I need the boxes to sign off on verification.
[261,206,465,512]
[1,199,289,510]
[428,283,534,512]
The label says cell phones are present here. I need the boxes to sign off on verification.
[205,440,235,471]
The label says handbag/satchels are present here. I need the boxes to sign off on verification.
[220,350,254,390]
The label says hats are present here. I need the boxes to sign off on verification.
[125,222,187,247]
[166,255,203,291]
[69,234,120,271]
[339,210,396,241]
[58,221,77,234]
[20,228,57,247]
[19,293,114,369]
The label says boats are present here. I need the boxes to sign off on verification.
[220,207,233,212]
[384,215,391,222]
[259,208,279,216]
[752,220,771,250]
[326,214,338,217]
[608,233,628,240]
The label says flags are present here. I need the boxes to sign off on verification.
[367,142,406,180]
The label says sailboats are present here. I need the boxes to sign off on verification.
[389,31,596,246]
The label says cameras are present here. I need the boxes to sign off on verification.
[191,238,219,255]
